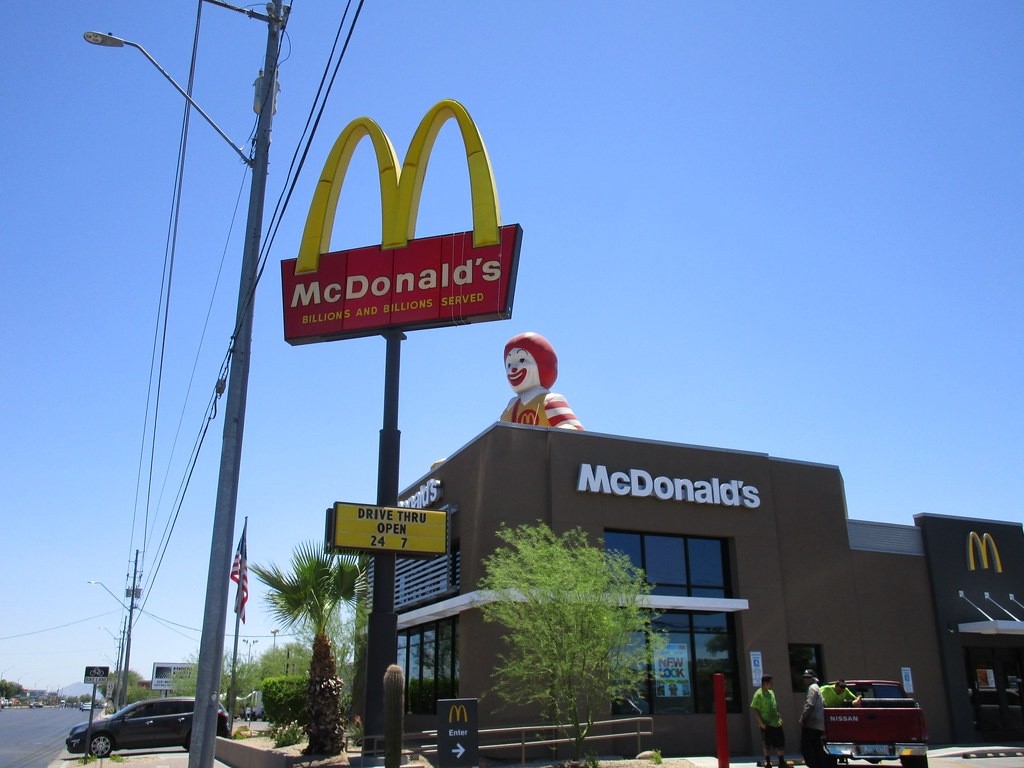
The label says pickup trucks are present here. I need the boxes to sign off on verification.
[819,679,929,768]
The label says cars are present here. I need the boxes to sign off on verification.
[80,702,92,712]
[967,688,1024,744]
[65,696,230,759]
[30,702,44,708]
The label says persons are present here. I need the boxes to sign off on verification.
[429,332,584,474]
[749,674,794,768]
[798,669,832,768]
[818,678,864,708]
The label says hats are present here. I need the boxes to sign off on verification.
[801,669,817,677]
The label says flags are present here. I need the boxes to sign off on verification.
[229,517,250,626]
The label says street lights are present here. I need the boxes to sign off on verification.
[100,626,125,714]
[84,30,281,767]
[87,580,138,709]
